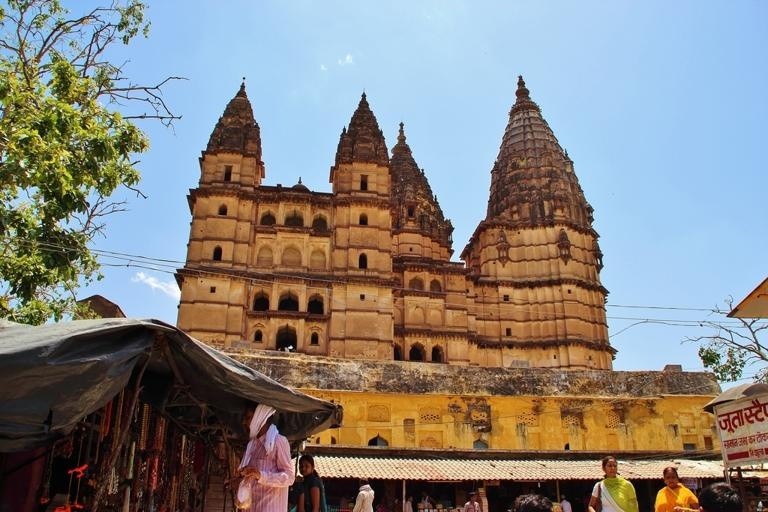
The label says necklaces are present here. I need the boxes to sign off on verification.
[38,394,198,512]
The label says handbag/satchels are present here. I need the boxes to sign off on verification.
[235,482,253,510]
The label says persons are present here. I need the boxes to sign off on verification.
[223,403,296,511]
[560,494,573,511]
[420,489,437,504]
[297,454,327,512]
[406,495,414,511]
[698,482,744,511]
[512,493,553,511]
[654,466,700,511]
[588,455,640,511]
[463,491,481,512]
[351,476,375,512]
